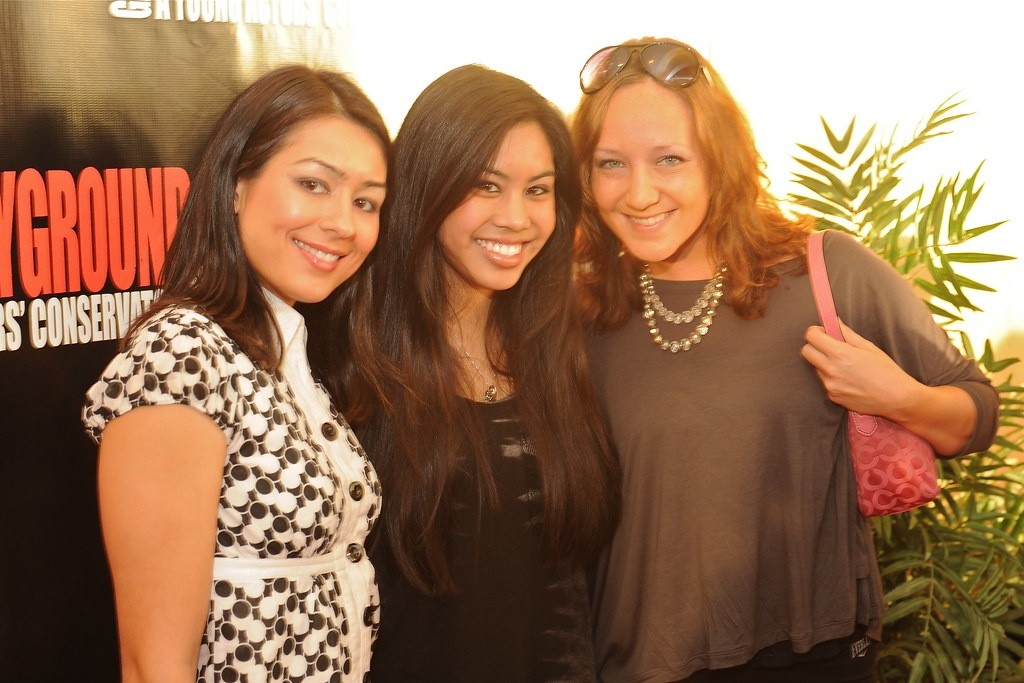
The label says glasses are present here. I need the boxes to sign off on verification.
[578,42,715,94]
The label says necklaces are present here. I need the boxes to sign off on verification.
[639,261,726,352]
[462,344,504,402]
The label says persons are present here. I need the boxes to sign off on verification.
[84,65,403,683]
[350,65,621,683]
[571,36,997,683]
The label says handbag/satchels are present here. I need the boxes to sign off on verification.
[807,230,940,518]
